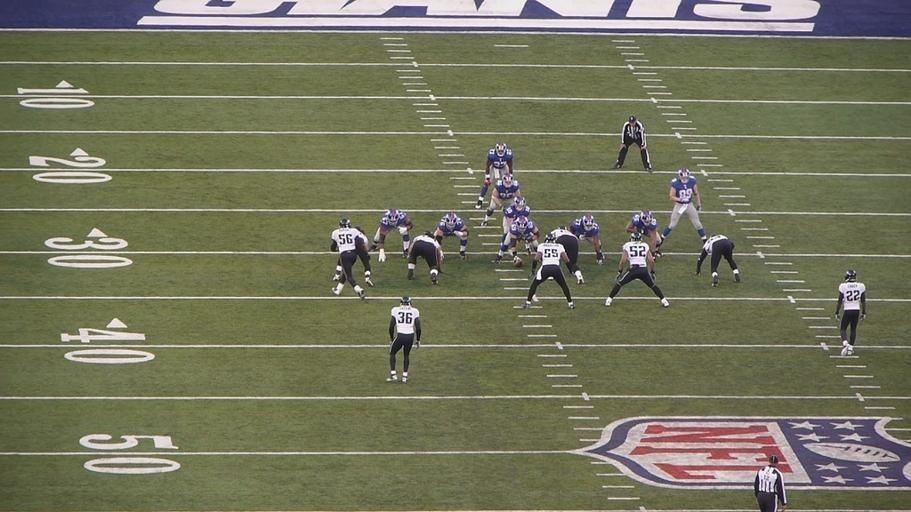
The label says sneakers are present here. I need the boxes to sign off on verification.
[605,300,611,305]
[532,294,538,302]
[358,288,365,301]
[495,254,503,263]
[386,374,408,383]
[661,297,670,307]
[704,239,707,243]
[407,271,414,279]
[841,344,854,356]
[735,279,740,283]
[403,249,408,257]
[526,301,530,304]
[368,245,378,252]
[475,205,481,208]
[332,287,342,295]
[577,275,584,284]
[366,277,375,287]
[568,302,575,309]
[481,221,488,226]
[432,273,438,284]
[712,274,719,287]
[333,274,340,281]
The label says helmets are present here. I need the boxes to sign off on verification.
[678,168,689,177]
[558,225,568,230]
[544,234,556,242]
[630,233,641,239]
[400,296,412,305]
[583,214,595,229]
[514,196,525,206]
[845,270,856,282]
[496,143,507,156]
[502,174,513,187]
[340,219,350,226]
[446,213,457,226]
[516,215,528,228]
[387,209,399,222]
[641,210,652,226]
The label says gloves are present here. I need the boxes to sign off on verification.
[651,273,656,281]
[398,227,407,233]
[440,250,444,260]
[454,231,463,237]
[414,340,420,349]
[615,272,621,281]
[860,314,866,321]
[379,249,386,262]
[835,314,840,322]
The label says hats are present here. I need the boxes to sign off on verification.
[629,116,635,122]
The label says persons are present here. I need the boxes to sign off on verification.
[475,141,604,309]
[433,213,469,260]
[615,115,654,170]
[333,228,375,287]
[386,296,422,384]
[755,455,787,511]
[625,209,662,263]
[604,232,670,307]
[408,230,443,286]
[656,167,707,251]
[695,234,741,286]
[368,209,414,263]
[835,269,867,355]
[330,219,368,301]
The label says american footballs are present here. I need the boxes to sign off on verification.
[515,259,521,268]
